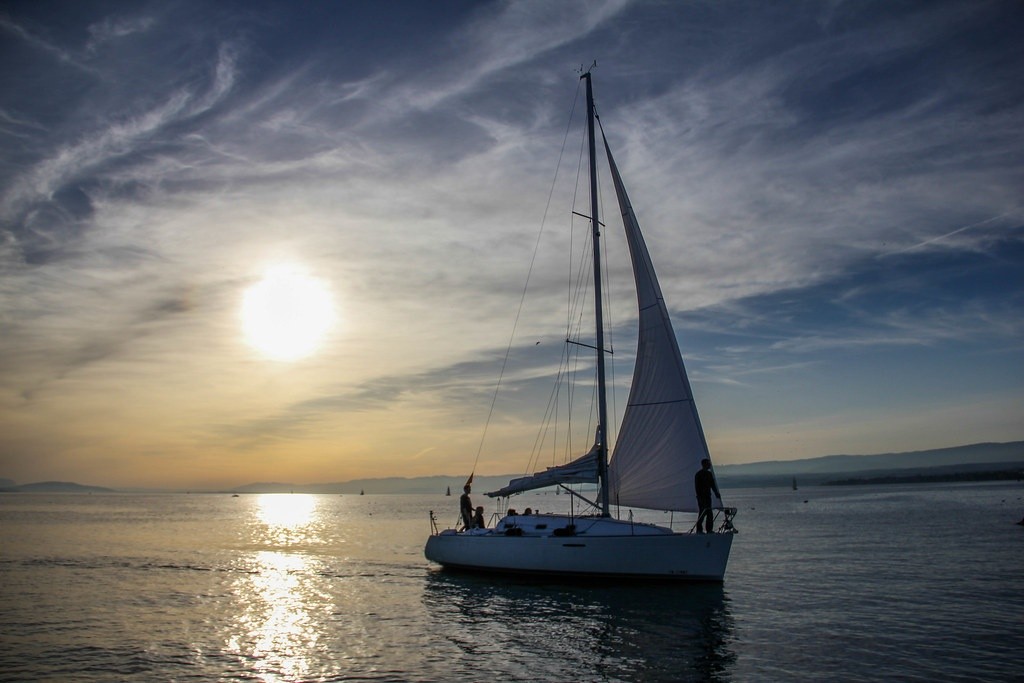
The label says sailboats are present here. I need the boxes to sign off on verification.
[424,56,741,592]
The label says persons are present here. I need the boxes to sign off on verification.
[694,458,721,534]
[458,485,485,532]
